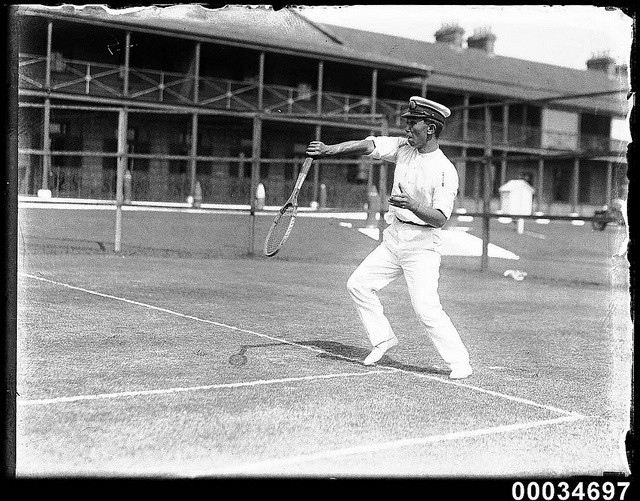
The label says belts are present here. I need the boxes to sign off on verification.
[395,215,436,228]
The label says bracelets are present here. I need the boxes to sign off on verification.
[413,201,422,214]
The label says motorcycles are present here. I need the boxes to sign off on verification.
[593,205,624,231]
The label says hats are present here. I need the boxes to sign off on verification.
[401,94,451,124]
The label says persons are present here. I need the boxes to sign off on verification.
[305,96,473,381]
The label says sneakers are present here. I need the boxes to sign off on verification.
[449,358,473,379]
[363,336,399,366]
